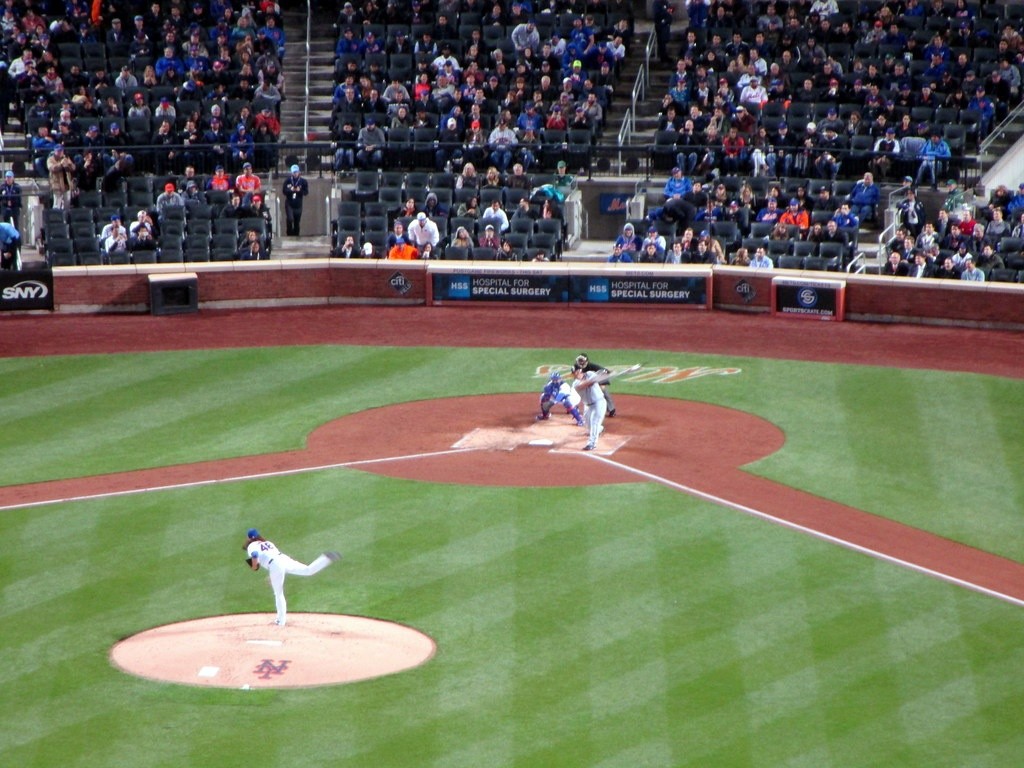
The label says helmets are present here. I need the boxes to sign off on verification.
[551,371,561,380]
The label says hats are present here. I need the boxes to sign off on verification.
[613,243,622,250]
[417,212,426,222]
[425,192,438,205]
[649,226,658,233]
[791,198,801,207]
[558,160,566,169]
[571,365,581,373]
[248,529,259,539]
[700,230,710,237]
[672,167,681,173]
[485,224,495,230]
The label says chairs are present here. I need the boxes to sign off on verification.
[335,0,1024,280]
[0,0,286,270]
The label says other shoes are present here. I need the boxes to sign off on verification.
[582,443,596,450]
[536,415,549,420]
[599,429,605,435]
[323,551,343,561]
[609,409,616,416]
[274,618,285,626]
[576,421,585,426]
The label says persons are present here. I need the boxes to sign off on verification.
[0,0,286,270]
[283,164,308,236]
[331,0,1024,282]
[536,372,585,426]
[571,353,616,450]
[242,529,338,626]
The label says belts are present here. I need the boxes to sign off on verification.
[268,552,282,564]
